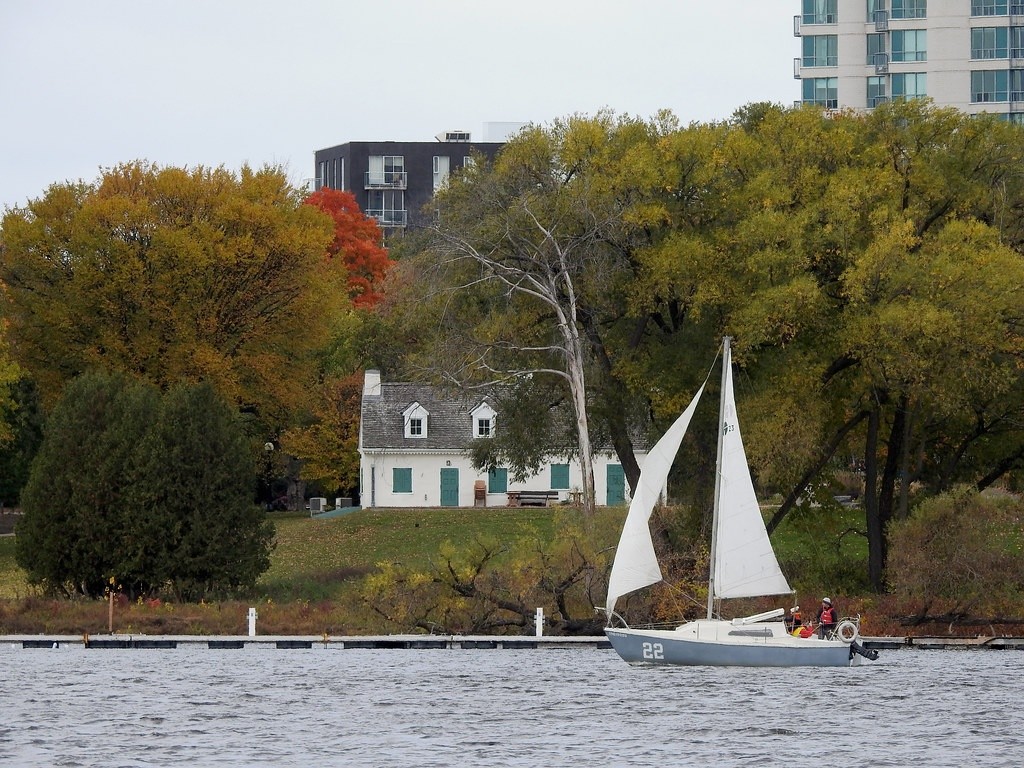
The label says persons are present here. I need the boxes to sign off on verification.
[816,598,838,640]
[785,606,812,637]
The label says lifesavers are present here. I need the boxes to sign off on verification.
[838,623,858,642]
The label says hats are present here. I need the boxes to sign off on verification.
[790,606,799,613]
[823,598,831,603]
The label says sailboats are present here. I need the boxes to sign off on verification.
[590,332,882,669]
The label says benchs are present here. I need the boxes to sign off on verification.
[508,490,558,506]
